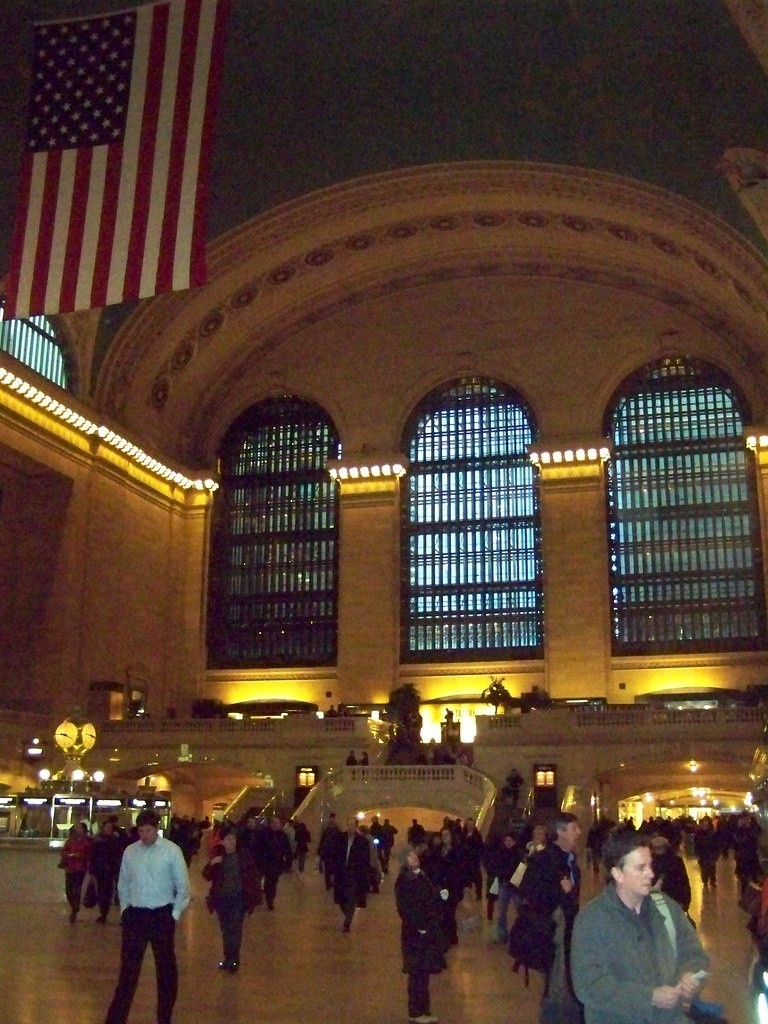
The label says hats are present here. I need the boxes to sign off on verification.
[396,846,415,869]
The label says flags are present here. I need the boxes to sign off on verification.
[1,0,233,322]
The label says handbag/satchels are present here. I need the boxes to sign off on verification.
[737,882,762,917]
[688,1000,727,1024]
[508,916,554,972]
[57,860,67,869]
[83,884,97,908]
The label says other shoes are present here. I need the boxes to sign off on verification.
[218,961,239,972]
[409,1014,438,1024]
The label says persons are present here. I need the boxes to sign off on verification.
[500,768,525,808]
[346,748,468,780]
[325,705,338,717]
[57,809,768,1024]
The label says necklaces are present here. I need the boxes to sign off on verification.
[629,896,642,915]
